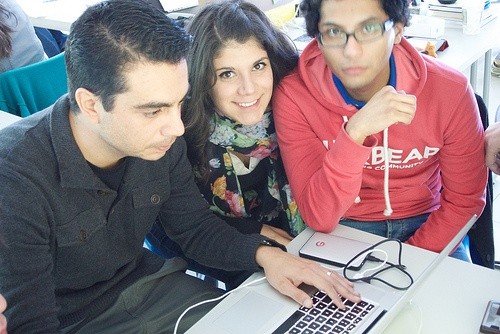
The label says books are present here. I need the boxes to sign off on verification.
[426,0,499,34]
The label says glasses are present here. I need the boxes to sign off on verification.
[315,17,394,48]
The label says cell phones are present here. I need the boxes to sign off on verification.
[298,231,376,272]
[479,300,499,334]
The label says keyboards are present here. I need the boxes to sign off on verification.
[268,284,387,334]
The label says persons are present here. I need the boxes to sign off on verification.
[179,0,309,254]
[480,55,500,177]
[0,0,49,74]
[269,0,489,264]
[0,290,15,334]
[0,0,364,334]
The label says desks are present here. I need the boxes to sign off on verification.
[183,225,500,334]
[407,24,500,109]
